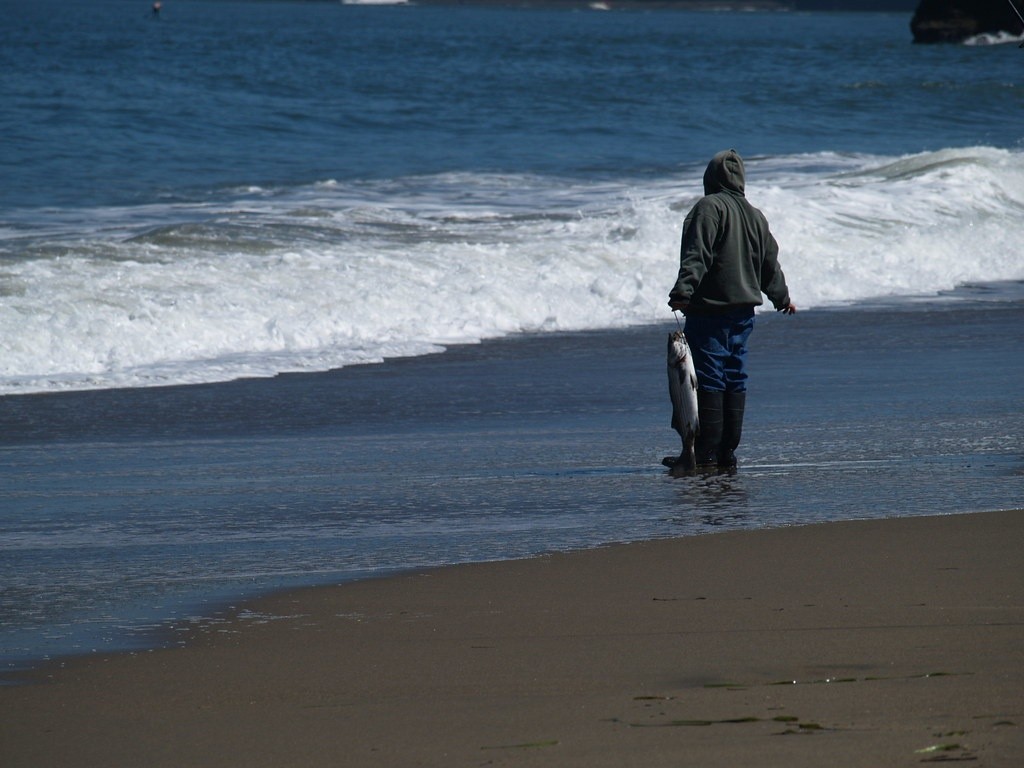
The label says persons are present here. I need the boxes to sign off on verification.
[660,148,795,470]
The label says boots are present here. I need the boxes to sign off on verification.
[718,392,746,473]
[662,390,723,473]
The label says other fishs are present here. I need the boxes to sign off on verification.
[667,332,699,470]
